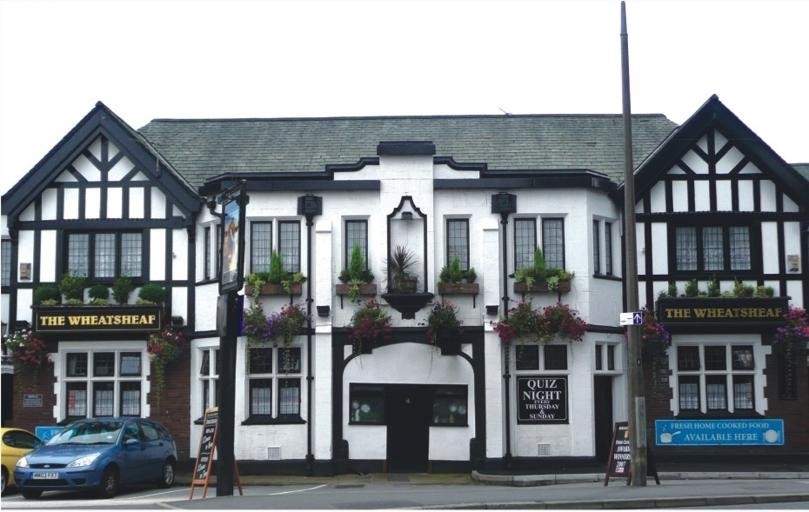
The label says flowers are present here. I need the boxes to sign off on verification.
[145,328,186,365]
[772,307,809,345]
[3,329,49,367]
[641,307,673,355]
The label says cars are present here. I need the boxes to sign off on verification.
[11,412,181,502]
[1,425,50,499]
